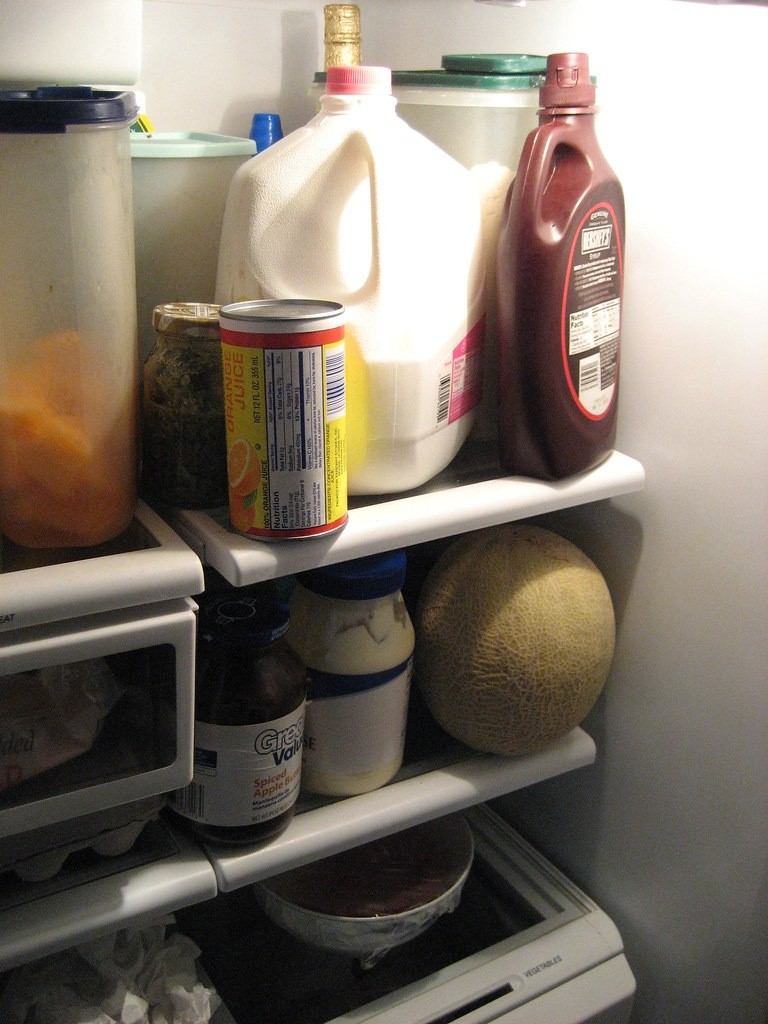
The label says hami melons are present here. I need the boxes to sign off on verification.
[416,525,614,755]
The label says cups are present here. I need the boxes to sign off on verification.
[1,86,140,550]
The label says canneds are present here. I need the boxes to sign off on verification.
[220,299,349,542]
[140,302,226,507]
[288,550,415,797]
[167,599,308,844]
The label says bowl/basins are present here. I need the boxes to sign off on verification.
[263,819,473,947]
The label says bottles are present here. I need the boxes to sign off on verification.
[498,53,626,479]
[325,4,361,74]
[214,66,486,495]
[308,72,326,114]
[286,552,414,795]
[155,600,308,851]
[143,302,228,509]
